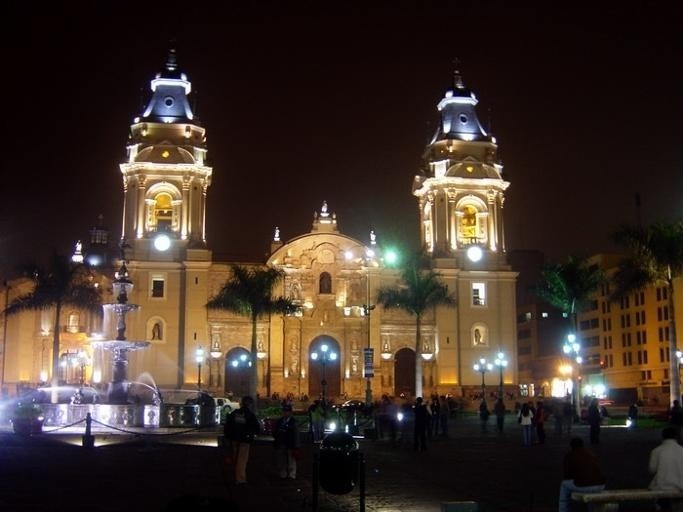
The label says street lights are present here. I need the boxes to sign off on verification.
[493,351,509,412]
[562,333,585,423]
[231,354,253,397]
[310,343,337,401]
[472,357,493,413]
[195,345,204,391]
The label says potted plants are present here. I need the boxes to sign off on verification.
[10,401,44,435]
[259,404,284,434]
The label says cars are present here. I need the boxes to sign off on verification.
[213,397,241,414]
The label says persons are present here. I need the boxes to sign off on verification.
[308,401,321,440]
[224,396,261,485]
[515,396,578,443]
[272,391,309,401]
[480,398,488,424]
[275,403,297,480]
[413,394,449,448]
[495,396,506,432]
[587,398,603,446]
[648,428,683,491]
[558,438,608,512]
[629,401,638,427]
[670,400,682,423]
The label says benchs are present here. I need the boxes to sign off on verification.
[568,487,682,509]
